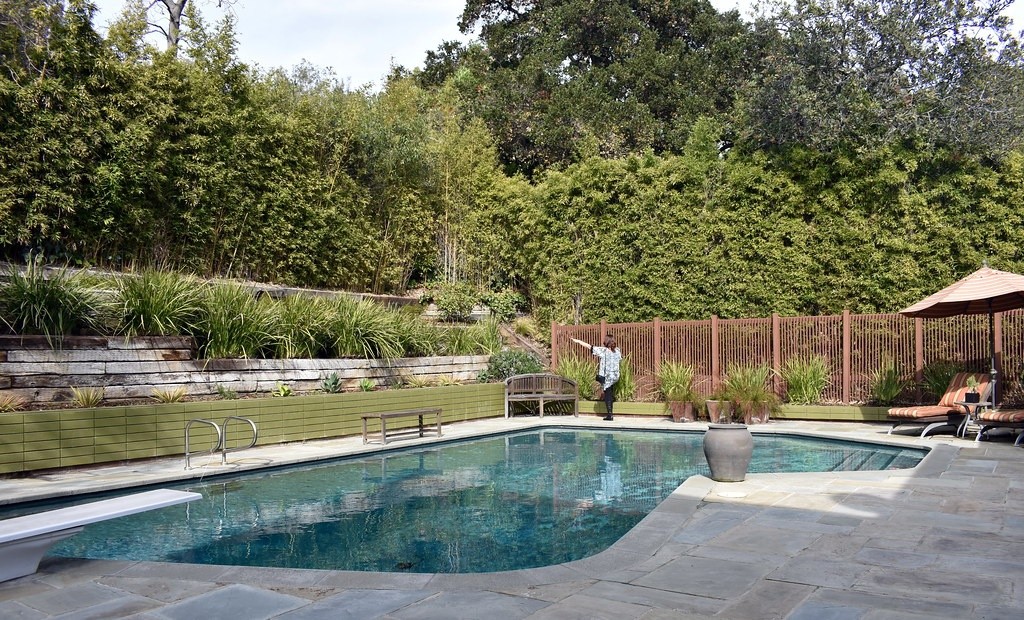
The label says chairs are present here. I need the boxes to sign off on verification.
[886,371,998,440]
[972,401,1024,448]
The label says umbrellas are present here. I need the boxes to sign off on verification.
[899,262,1024,411]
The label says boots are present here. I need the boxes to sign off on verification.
[603,401,613,421]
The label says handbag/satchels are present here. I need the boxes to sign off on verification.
[596,375,605,384]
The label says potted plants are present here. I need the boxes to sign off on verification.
[705,392,736,424]
[651,359,704,424]
[720,360,786,425]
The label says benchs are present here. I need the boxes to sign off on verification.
[503,373,579,418]
[360,407,443,445]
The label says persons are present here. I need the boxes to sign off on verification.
[569,335,622,420]
